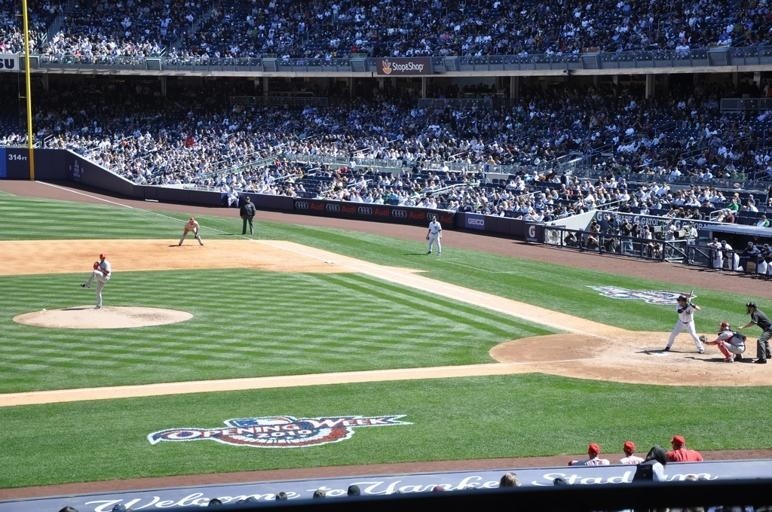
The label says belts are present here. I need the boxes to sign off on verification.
[680,320,692,324]
[735,345,745,347]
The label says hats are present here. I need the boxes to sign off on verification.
[745,302,757,309]
[100,254,106,260]
[671,434,685,448]
[624,441,635,452]
[588,443,600,455]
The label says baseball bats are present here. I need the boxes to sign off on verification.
[688,290,693,306]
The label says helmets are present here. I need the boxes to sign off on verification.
[677,295,687,301]
[719,321,730,331]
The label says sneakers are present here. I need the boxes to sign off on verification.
[80,284,89,288]
[754,358,767,364]
[721,357,743,363]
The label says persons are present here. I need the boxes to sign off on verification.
[738,298,772,367]
[631,444,669,482]
[661,290,705,360]
[567,443,612,467]
[616,441,645,464]
[76,249,113,313]
[697,318,746,364]
[665,435,704,461]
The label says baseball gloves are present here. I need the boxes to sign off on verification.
[93,262,100,270]
[700,336,707,342]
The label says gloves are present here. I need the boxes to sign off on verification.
[689,302,696,309]
[677,304,689,313]
